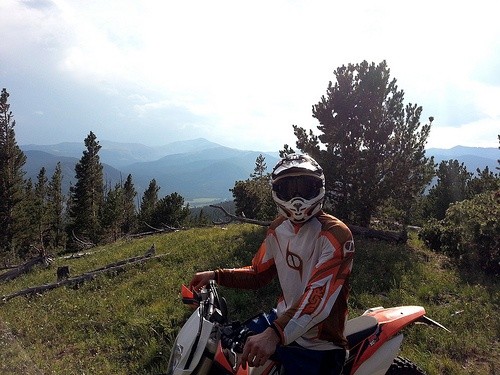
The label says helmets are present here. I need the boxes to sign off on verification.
[269,153,326,224]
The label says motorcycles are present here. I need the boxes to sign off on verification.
[166,279,451,375]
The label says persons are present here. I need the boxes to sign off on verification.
[188,154,354,375]
